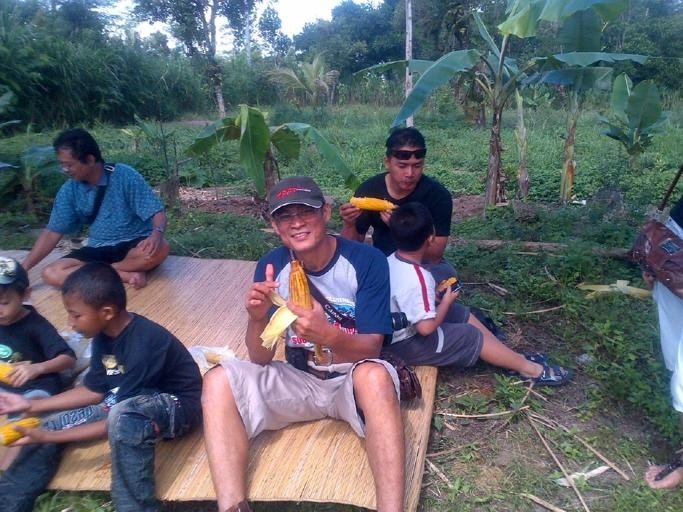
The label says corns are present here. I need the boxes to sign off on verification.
[350,196,398,211]
[286,261,313,311]
[0,418,39,445]
[437,276,456,294]
[0,361,17,386]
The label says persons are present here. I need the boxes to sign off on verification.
[345,126,452,269]
[0,254,77,512]
[19,127,171,289]
[643,196,682,497]
[198,176,403,511]
[9,264,202,512]
[382,202,569,386]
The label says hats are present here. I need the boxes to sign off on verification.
[0,256,28,285]
[269,176,324,215]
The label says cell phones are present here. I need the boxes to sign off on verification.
[438,281,462,299]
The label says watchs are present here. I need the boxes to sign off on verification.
[154,226,164,233]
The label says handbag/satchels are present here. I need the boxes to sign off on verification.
[379,349,421,402]
[628,217,683,298]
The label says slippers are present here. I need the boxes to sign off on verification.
[647,460,683,491]
[521,363,569,386]
[509,351,547,376]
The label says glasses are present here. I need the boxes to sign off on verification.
[274,207,315,221]
[391,149,426,159]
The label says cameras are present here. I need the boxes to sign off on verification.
[382,312,408,346]
